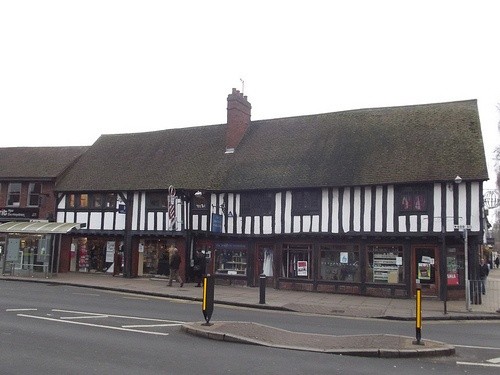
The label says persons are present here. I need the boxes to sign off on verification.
[480,259,489,295]
[194,248,207,287]
[496,257,499,268]
[166,248,184,287]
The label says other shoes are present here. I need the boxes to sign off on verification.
[166,284,172,286]
[179,281,183,287]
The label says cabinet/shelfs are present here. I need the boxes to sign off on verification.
[372,247,404,283]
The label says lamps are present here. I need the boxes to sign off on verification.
[194,191,202,198]
[454,175,462,184]
